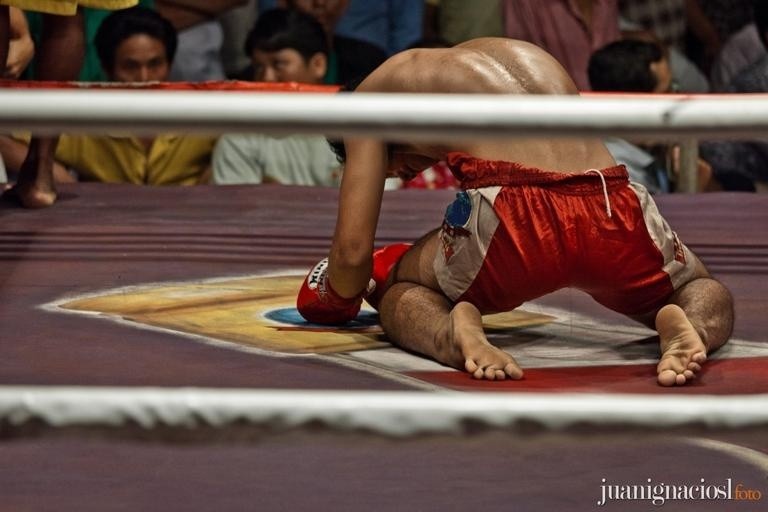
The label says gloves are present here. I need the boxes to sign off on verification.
[361,241,414,312]
[295,256,377,327]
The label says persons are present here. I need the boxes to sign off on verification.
[299,34,734,387]
